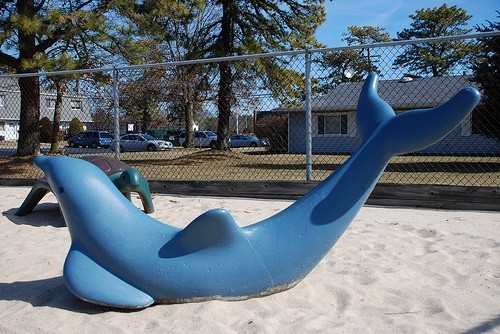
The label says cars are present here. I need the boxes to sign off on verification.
[110,134,173,151]
[228,134,269,147]
[178,131,217,148]
[67,131,113,149]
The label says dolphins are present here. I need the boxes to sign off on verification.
[32,72,481,308]
